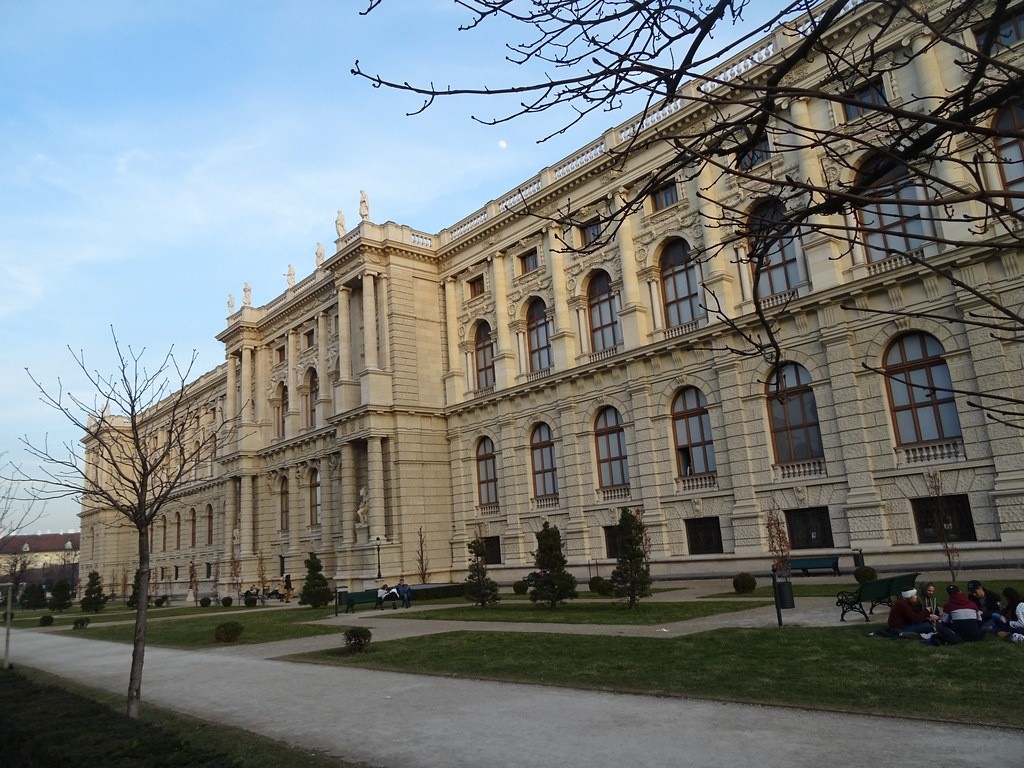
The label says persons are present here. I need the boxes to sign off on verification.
[316,241,325,266]
[284,264,295,287]
[227,294,235,314]
[887,579,1024,645]
[335,210,346,237]
[243,282,253,307]
[359,190,369,220]
[397,579,410,608]
[377,584,397,609]
[280,574,292,602]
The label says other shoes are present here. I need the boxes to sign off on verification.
[1010,633,1024,642]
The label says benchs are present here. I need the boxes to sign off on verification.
[786,556,842,578]
[346,589,411,614]
[836,573,923,623]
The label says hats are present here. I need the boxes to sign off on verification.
[946,584,960,594]
[967,580,981,591]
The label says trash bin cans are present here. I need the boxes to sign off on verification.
[774,571,795,609]
[338,591,348,606]
[263,586,270,595]
